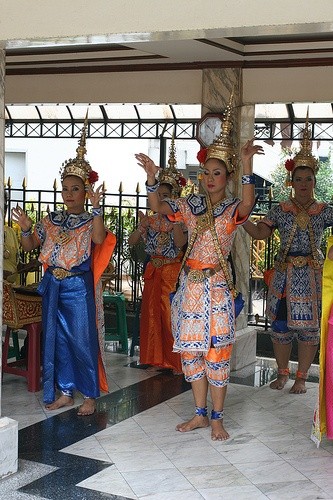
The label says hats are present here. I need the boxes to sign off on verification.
[59,157,99,182]
[197,137,241,175]
[291,149,321,176]
[157,166,187,197]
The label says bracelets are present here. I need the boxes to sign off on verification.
[92,207,102,217]
[20,228,32,238]
[241,175,257,185]
[145,180,161,193]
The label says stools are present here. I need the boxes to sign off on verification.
[103,291,129,355]
[2,322,45,392]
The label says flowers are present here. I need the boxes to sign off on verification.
[179,177,186,186]
[87,171,98,186]
[197,147,207,164]
[285,159,294,171]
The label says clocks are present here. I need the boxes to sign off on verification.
[196,112,225,149]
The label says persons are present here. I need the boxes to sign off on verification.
[10,157,115,415]
[133,138,267,441]
[240,153,333,395]
[127,168,188,375]
